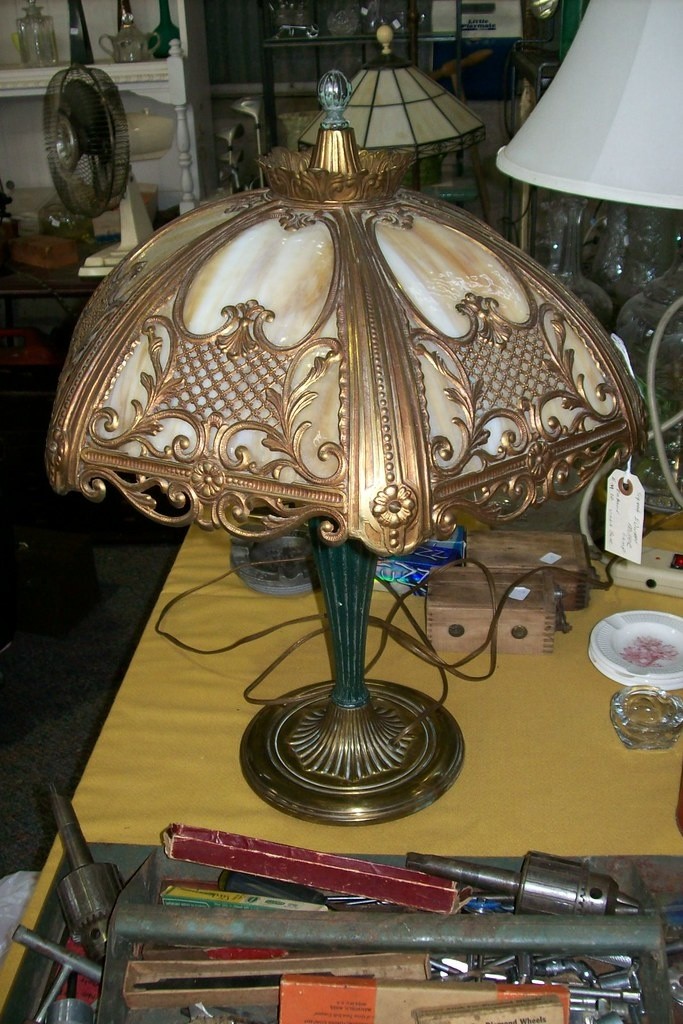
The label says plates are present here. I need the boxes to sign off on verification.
[588,610,683,691]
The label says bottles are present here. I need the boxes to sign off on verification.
[148,0,180,58]
[15,5,58,67]
[535,196,683,406]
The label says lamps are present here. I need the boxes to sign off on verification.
[299,18,487,194]
[495,0,683,211]
[42,68,649,827]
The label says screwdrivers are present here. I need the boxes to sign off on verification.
[218,865,464,977]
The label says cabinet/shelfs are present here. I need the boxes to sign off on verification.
[0,0,219,218]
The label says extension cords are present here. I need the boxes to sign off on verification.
[607,546,683,597]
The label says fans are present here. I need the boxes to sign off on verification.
[43,63,177,277]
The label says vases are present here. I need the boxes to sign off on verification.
[540,198,682,511]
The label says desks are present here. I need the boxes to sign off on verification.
[0,503,683,1024]
[0,204,190,548]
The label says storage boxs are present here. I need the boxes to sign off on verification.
[10,233,79,270]
